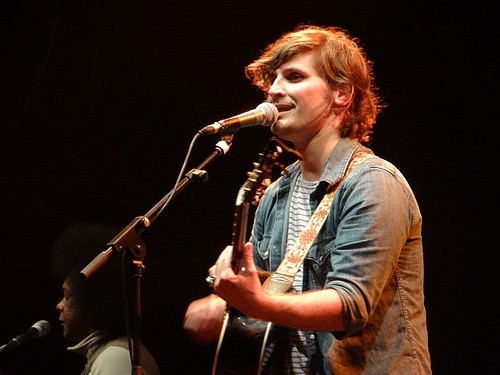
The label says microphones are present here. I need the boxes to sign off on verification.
[198,102,279,136]
[7,319,50,349]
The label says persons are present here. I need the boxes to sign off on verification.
[55,262,162,374]
[183,24,435,375]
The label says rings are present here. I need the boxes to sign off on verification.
[204,274,217,284]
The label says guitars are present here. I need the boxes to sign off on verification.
[211,145,290,375]
